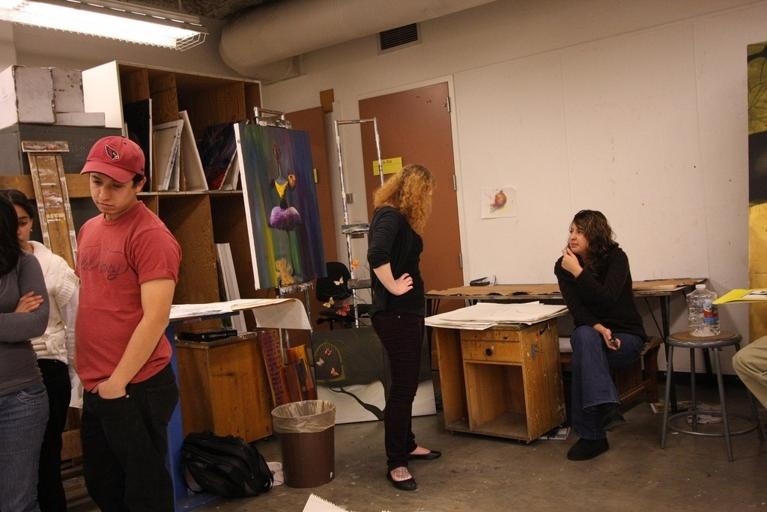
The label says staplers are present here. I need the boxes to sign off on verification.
[470,277,490,286]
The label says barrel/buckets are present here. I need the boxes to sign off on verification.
[686,284,720,336]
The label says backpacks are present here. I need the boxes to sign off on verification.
[179,429,274,499]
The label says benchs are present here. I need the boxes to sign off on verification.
[559,336,658,428]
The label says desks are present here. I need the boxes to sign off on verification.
[428,278,710,410]
[174,331,276,446]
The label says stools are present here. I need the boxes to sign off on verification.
[660,329,762,463]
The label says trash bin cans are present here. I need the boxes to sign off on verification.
[271,400,336,488]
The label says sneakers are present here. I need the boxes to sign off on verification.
[568,437,609,461]
[598,404,625,432]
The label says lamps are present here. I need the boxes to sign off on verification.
[0,1,211,53]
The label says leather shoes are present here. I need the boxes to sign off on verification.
[406,450,442,460]
[387,470,417,490]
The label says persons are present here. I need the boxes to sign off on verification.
[3,189,80,512]
[0,196,51,512]
[731,335,767,409]
[554,209,647,460]
[75,135,183,512]
[366,164,437,490]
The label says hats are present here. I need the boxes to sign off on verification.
[79,136,146,184]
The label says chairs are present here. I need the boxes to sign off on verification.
[316,261,372,330]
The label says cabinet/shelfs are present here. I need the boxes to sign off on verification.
[79,60,264,337]
[426,302,567,442]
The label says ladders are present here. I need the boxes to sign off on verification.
[334,117,384,328]
